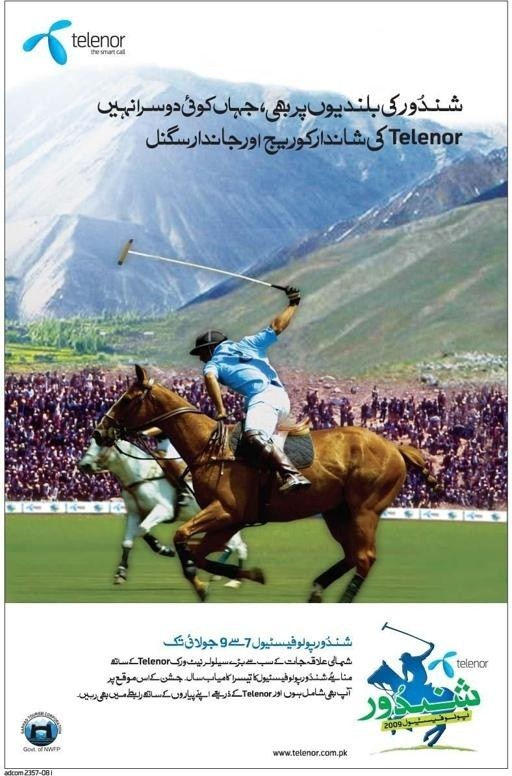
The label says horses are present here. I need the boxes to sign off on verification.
[90,362,444,603]
[77,433,250,591]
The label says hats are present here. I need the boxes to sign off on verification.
[190,330,228,355]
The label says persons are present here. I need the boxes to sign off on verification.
[189,286,312,494]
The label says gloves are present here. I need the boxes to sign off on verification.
[284,285,301,306]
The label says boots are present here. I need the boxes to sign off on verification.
[243,429,312,492]
